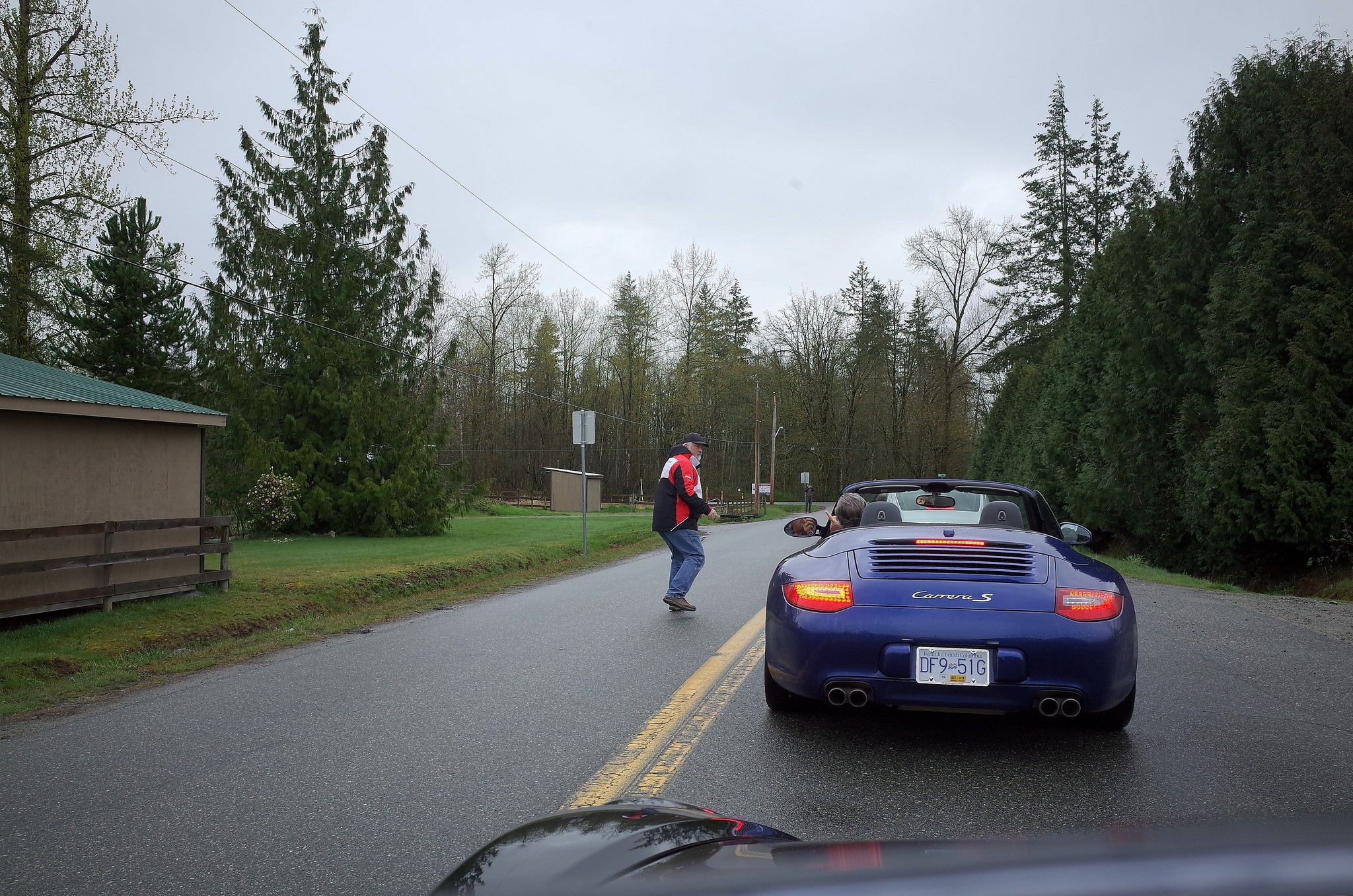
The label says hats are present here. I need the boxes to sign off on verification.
[683,432,709,448]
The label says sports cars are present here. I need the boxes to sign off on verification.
[761,477,1140,732]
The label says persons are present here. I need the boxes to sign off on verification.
[804,483,815,513]
[917,496,934,506]
[789,518,815,535]
[650,431,719,611]
[824,493,867,531]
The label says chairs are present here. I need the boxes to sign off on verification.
[860,501,903,526]
[978,500,1024,529]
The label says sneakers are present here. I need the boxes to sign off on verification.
[663,596,697,611]
[669,605,683,611]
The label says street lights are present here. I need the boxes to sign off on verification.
[770,426,783,504]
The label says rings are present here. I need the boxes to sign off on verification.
[830,521,832,523]
[804,523,807,525]
